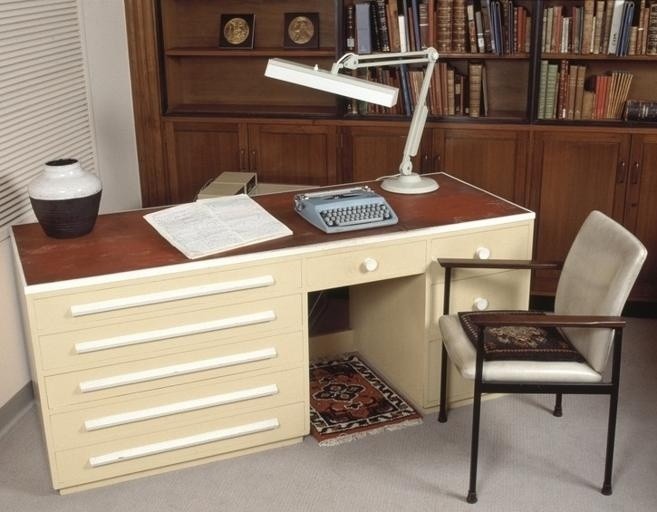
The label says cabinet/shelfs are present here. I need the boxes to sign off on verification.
[124,0,657,315]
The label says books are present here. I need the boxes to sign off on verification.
[344,1,656,118]
[143,192,293,260]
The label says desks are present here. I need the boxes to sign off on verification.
[9,171,536,495]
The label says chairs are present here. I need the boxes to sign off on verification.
[438,210,648,503]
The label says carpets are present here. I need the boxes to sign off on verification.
[310,351,424,446]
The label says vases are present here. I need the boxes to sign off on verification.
[28,158,102,239]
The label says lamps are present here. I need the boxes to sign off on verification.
[264,46,440,194]
